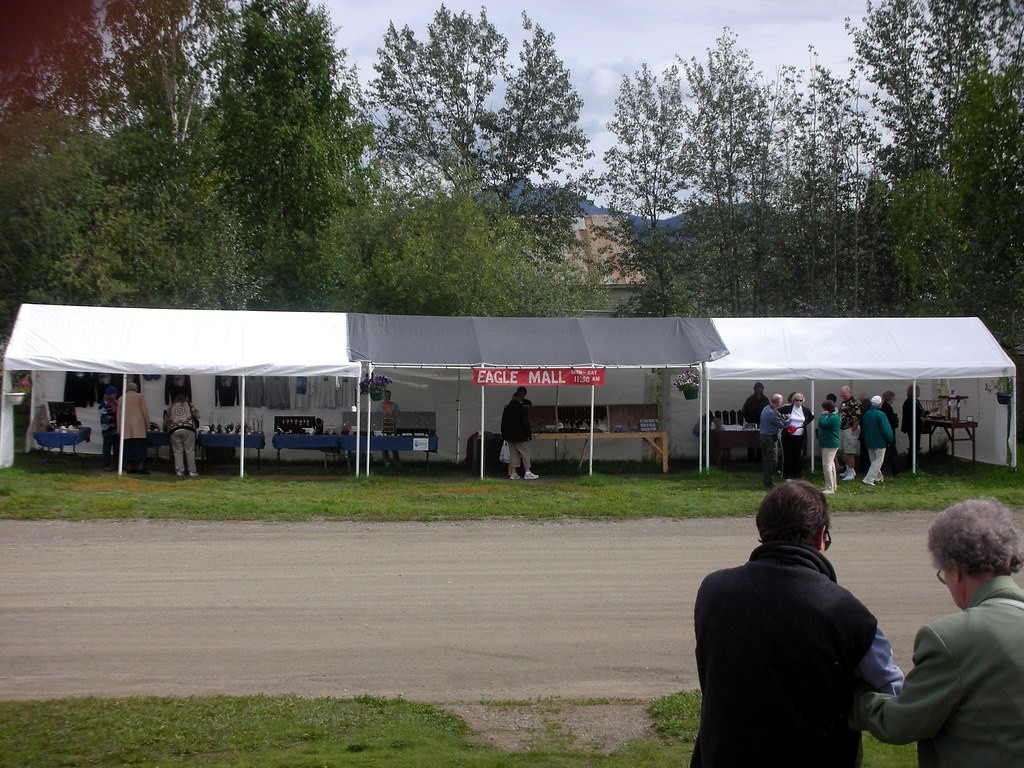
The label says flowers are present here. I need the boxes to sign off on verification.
[12,378,33,394]
[674,370,702,391]
[984,376,1015,395]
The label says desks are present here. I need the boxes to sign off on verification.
[524,428,672,473]
[271,432,342,467]
[702,425,809,467]
[200,432,266,468]
[124,431,183,472]
[341,432,443,467]
[920,417,979,464]
[33,425,92,463]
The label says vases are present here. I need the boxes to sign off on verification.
[998,395,1011,405]
[6,392,25,405]
[681,385,700,400]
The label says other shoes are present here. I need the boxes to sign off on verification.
[189,472,199,477]
[822,490,834,494]
[127,468,137,473]
[840,471,857,476]
[177,472,184,477]
[862,479,875,486]
[137,468,150,474]
[842,476,854,480]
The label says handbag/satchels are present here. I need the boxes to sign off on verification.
[188,402,199,428]
[499,440,511,463]
[786,426,804,434]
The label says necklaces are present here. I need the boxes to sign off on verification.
[315,420,323,427]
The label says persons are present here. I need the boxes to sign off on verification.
[858,390,900,486]
[516,386,533,406]
[501,393,539,480]
[376,390,401,469]
[99,385,120,473]
[777,391,815,479]
[818,386,861,494]
[760,394,791,488]
[116,382,152,475]
[164,393,202,478]
[901,384,939,471]
[741,381,771,472]
[689,477,905,768]
[849,497,1024,768]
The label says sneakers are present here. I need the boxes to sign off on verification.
[510,473,520,480]
[524,473,539,479]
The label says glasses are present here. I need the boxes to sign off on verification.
[825,530,832,550]
[795,400,803,402]
[937,568,945,585]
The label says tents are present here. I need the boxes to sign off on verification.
[1,302,729,480]
[704,317,1017,475]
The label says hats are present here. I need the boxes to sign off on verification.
[105,387,118,395]
[871,396,883,405]
